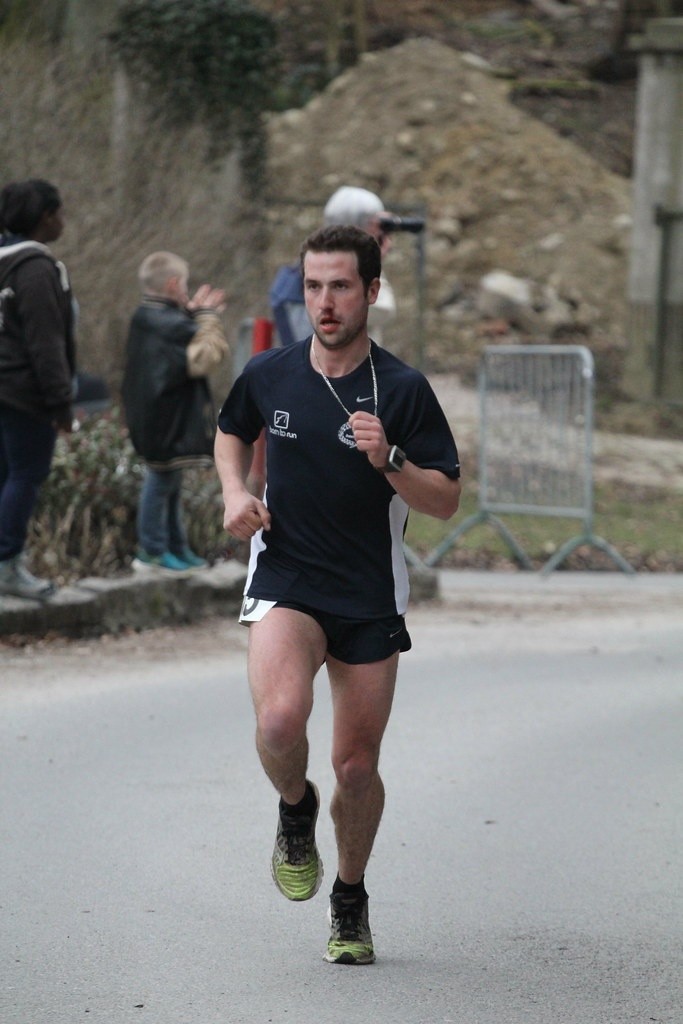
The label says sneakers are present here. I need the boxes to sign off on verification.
[325,888,376,964]
[271,779,324,902]
[170,545,210,570]
[130,544,194,579]
[0,553,57,599]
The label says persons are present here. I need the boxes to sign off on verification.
[0,178,78,598]
[119,253,228,575]
[238,185,409,627]
[212,224,462,963]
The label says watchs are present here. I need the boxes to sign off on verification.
[374,445,406,473]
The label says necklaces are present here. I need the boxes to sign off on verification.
[312,334,378,417]
